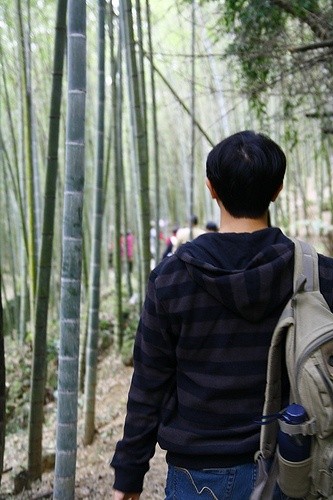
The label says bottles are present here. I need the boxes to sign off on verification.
[254,405,312,461]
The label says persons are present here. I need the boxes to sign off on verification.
[150,221,158,270]
[205,221,219,233]
[112,230,134,273]
[111,130,333,500]
[173,216,206,253]
[161,229,178,259]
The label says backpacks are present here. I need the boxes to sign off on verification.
[250,236,333,500]
